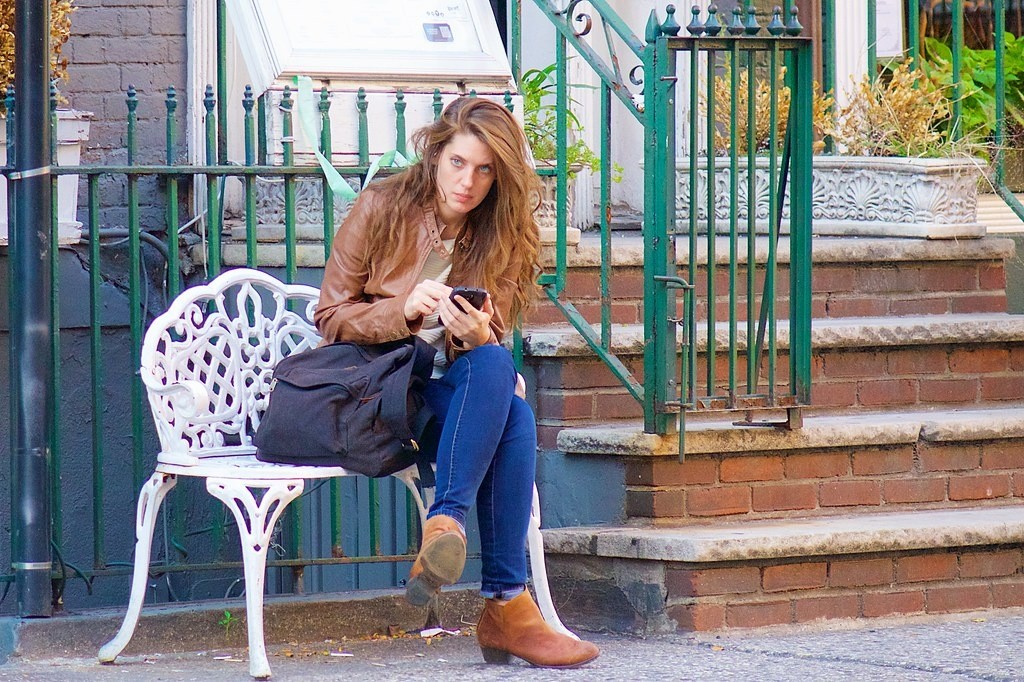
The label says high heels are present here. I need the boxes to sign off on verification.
[477,585,600,668]
[405,515,467,606]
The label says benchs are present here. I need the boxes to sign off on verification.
[96,267,582,682]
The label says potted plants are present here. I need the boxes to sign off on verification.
[0,0,92,246]
[880,31,1024,194]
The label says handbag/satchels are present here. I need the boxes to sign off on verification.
[252,335,442,488]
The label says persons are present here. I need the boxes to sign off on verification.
[312,93,600,671]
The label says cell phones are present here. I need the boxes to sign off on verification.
[438,286,487,327]
